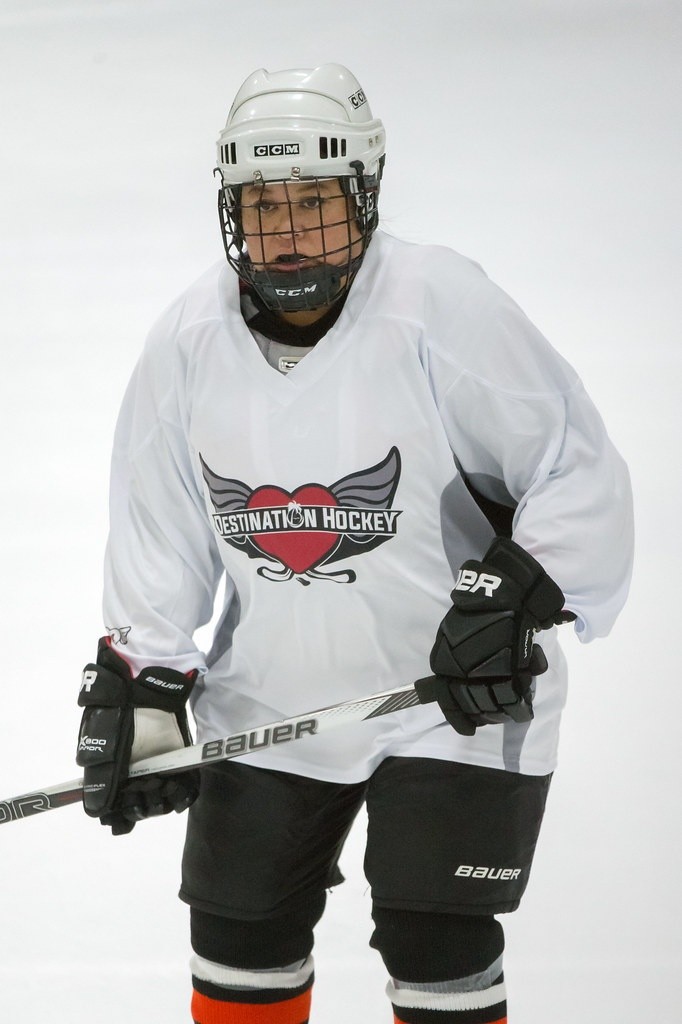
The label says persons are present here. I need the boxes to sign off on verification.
[73,53,634,1024]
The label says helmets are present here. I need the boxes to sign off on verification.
[214,64,386,312]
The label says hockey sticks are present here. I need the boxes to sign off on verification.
[1,641,552,825]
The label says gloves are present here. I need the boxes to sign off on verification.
[76,634,202,836]
[427,535,564,737]
